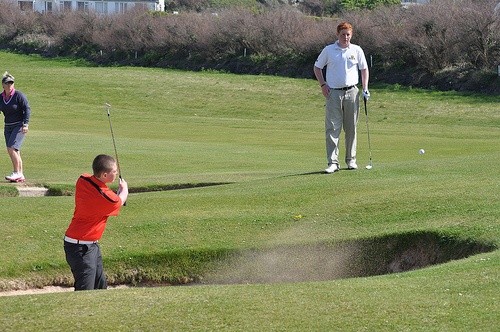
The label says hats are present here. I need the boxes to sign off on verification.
[2,77,14,83]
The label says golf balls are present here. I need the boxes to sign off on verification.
[419,148,425,154]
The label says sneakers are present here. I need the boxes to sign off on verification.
[348,163,357,170]
[324,164,340,173]
[5,172,25,182]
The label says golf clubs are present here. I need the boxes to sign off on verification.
[363,91,373,169]
[106,103,126,207]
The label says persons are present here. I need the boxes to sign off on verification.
[63,154,128,291]
[313,22,370,174]
[0,71,31,183]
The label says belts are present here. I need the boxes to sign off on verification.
[330,85,357,91]
[64,236,98,244]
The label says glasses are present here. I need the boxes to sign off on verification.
[5,81,14,85]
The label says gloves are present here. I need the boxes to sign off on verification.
[362,90,370,100]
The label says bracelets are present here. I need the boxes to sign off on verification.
[321,83,326,87]
